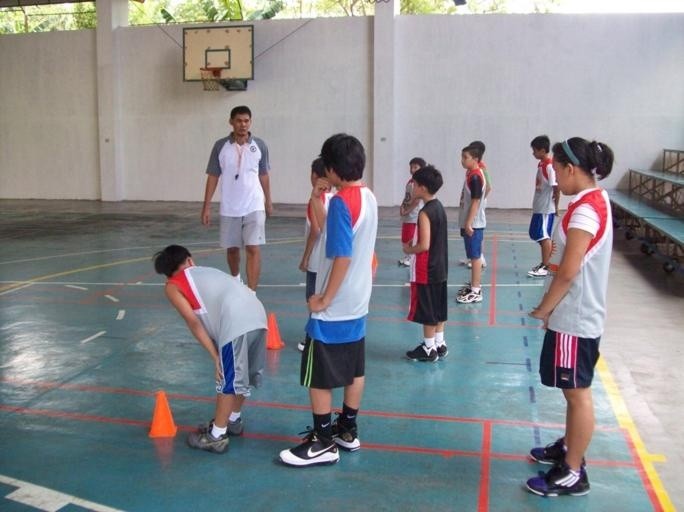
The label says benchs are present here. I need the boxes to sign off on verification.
[607,147,684,273]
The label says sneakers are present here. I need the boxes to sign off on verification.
[456,282,484,303]
[329,415,362,453]
[526,261,548,277]
[405,340,449,363]
[525,460,591,498]
[186,418,231,454]
[278,428,341,468]
[529,436,587,472]
[397,255,411,268]
[459,254,487,269]
[197,415,243,436]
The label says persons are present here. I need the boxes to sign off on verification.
[278,133,379,467]
[154,243,268,453]
[297,157,337,352]
[401,141,492,362]
[527,135,562,278]
[201,105,273,298]
[525,138,613,498]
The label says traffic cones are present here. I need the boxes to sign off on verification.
[267,345,282,377]
[150,431,174,470]
[146,388,180,440]
[264,310,286,351]
